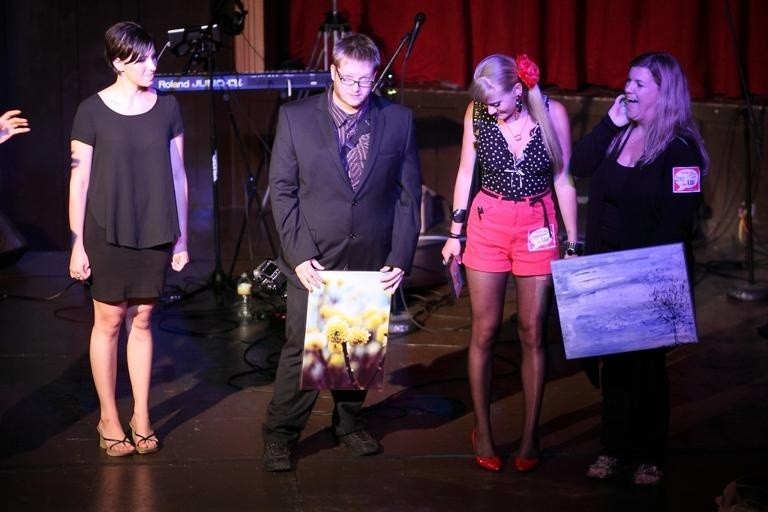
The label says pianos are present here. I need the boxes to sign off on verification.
[149,66,332,95]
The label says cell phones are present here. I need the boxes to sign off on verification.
[620,98,626,105]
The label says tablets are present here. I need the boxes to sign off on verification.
[445,255,465,302]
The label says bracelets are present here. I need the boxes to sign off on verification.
[449,230,459,237]
[566,239,583,254]
[450,208,466,223]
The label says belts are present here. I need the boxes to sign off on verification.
[481,188,551,202]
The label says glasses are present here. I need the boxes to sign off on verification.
[335,69,376,87]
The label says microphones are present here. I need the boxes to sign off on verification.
[405,13,427,60]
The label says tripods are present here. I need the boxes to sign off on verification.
[160,42,239,312]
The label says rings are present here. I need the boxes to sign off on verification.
[76,275,82,278]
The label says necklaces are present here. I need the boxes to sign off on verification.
[626,121,648,145]
[501,113,534,142]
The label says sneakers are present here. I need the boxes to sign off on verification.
[587,455,618,478]
[634,463,659,483]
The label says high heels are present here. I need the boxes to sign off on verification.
[129,421,160,454]
[472,430,502,470]
[515,458,539,471]
[96,426,135,457]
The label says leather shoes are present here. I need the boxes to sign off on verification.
[265,442,291,472]
[343,429,380,456]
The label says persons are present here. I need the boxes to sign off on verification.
[260,31,423,472]
[65,21,192,457]
[0,106,31,146]
[439,53,578,473]
[572,50,711,486]
